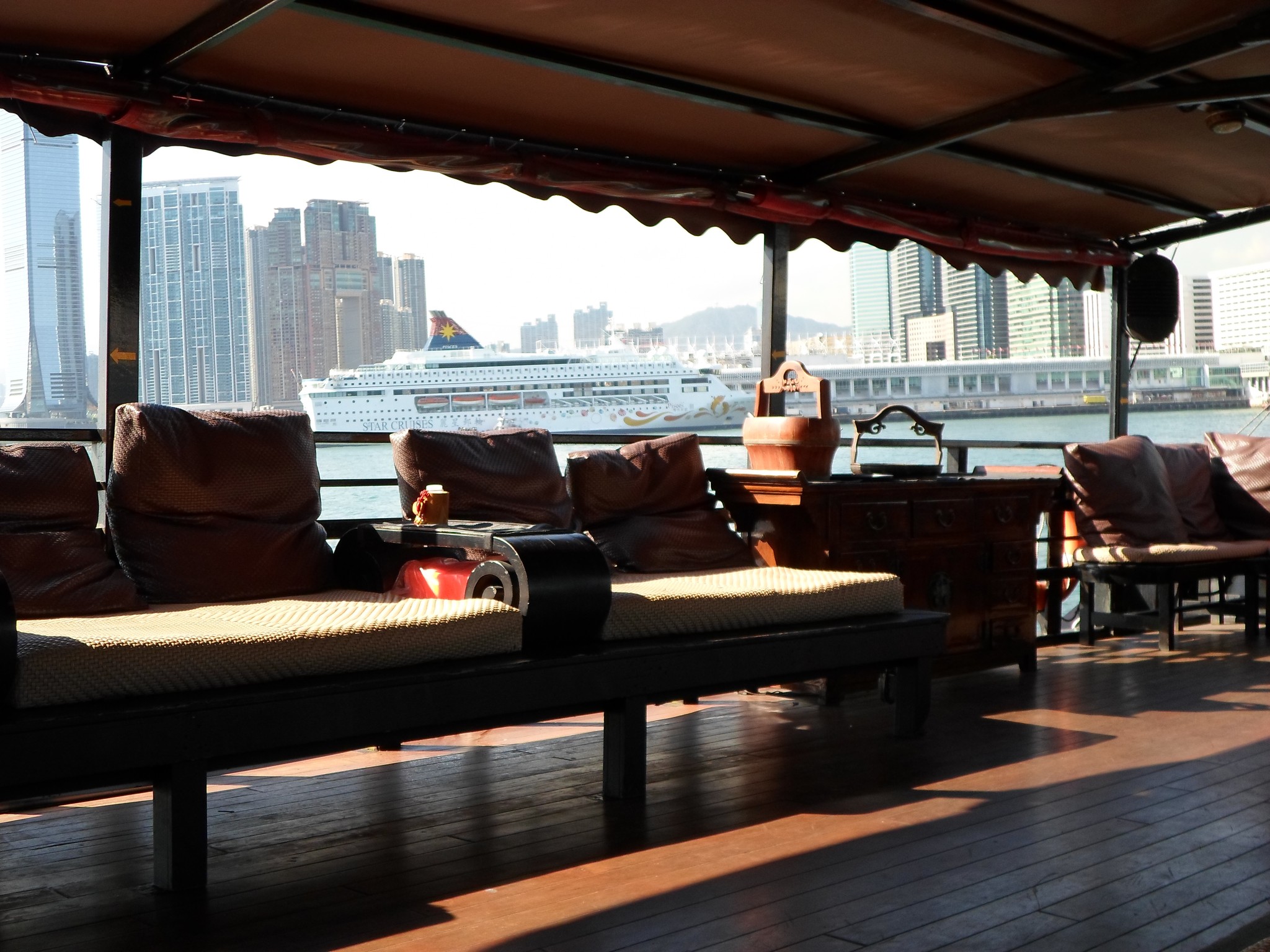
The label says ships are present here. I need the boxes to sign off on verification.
[296,312,755,433]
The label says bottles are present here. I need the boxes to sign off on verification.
[426,484,443,492]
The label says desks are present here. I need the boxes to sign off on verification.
[708,469,1063,706]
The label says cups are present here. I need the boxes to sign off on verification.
[413,491,449,527]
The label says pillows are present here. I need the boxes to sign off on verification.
[388,429,572,531]
[105,403,336,605]
[1062,434,1192,545]
[1204,432,1270,539]
[566,434,749,573]
[0,442,142,617]
[1156,443,1219,541]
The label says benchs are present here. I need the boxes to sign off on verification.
[0,404,569,894]
[1047,432,1270,651]
[387,428,951,810]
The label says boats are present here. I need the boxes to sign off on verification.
[2,0,1269,951]
[417,397,448,408]
[452,396,485,406]
[525,399,545,404]
[488,395,521,405]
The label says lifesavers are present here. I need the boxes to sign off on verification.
[1035,511,1087,613]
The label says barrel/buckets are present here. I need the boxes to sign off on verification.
[743,360,841,480]
